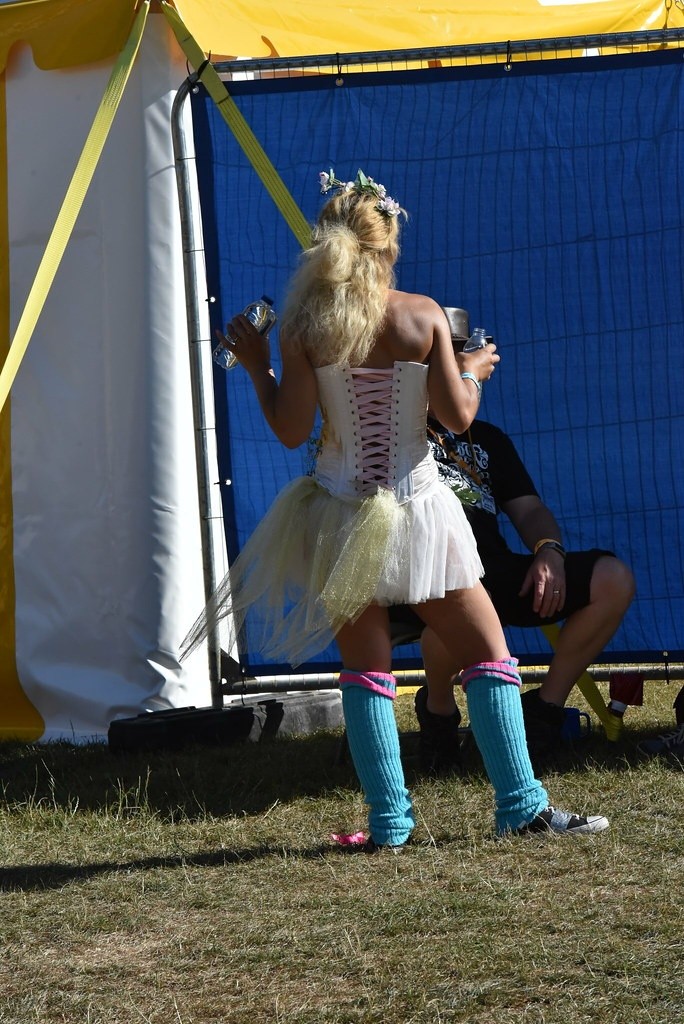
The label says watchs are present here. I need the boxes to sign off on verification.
[537,542,569,561]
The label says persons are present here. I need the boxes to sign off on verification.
[392,402,637,777]
[216,168,611,855]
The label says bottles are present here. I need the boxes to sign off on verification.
[213,295,273,370]
[463,328,487,408]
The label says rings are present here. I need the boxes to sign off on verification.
[233,337,240,344]
[553,590,561,594]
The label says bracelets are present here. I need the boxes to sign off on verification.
[461,372,484,402]
[533,537,565,554]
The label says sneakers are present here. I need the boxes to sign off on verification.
[363,836,416,854]
[495,804,610,841]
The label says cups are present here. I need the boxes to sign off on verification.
[558,708,591,743]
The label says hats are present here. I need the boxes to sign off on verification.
[440,306,493,343]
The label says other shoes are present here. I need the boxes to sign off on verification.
[521,688,564,778]
[415,686,464,779]
